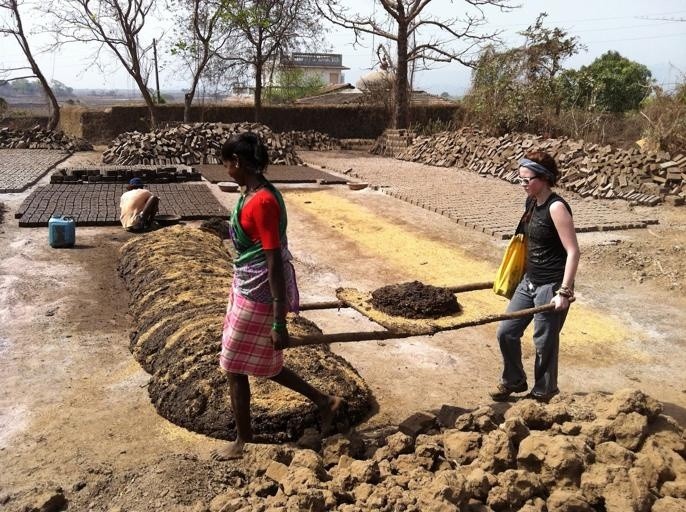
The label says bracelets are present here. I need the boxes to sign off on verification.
[557,285,574,302]
[270,321,287,332]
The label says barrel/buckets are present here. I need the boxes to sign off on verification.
[49,214,75,250]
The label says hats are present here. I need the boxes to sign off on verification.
[129,177,147,186]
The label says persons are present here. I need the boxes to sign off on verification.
[207,132,347,463]
[488,152,580,406]
[119,177,160,234]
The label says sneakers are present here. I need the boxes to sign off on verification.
[523,387,559,404]
[488,377,528,402]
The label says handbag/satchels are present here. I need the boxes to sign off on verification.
[493,198,537,300]
